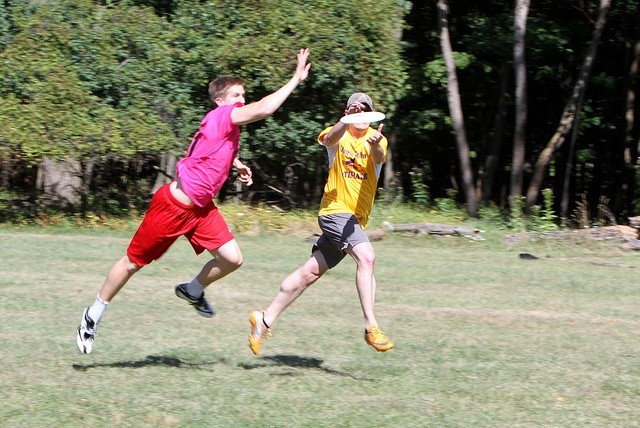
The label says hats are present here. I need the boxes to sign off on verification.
[347,93,373,111]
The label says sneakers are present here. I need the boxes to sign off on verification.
[76,304,101,354]
[249,310,272,356]
[175,283,214,318]
[365,325,394,352]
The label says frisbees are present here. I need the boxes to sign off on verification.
[340,112,385,124]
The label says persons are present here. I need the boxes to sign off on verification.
[75,48,312,354]
[249,90,395,354]
